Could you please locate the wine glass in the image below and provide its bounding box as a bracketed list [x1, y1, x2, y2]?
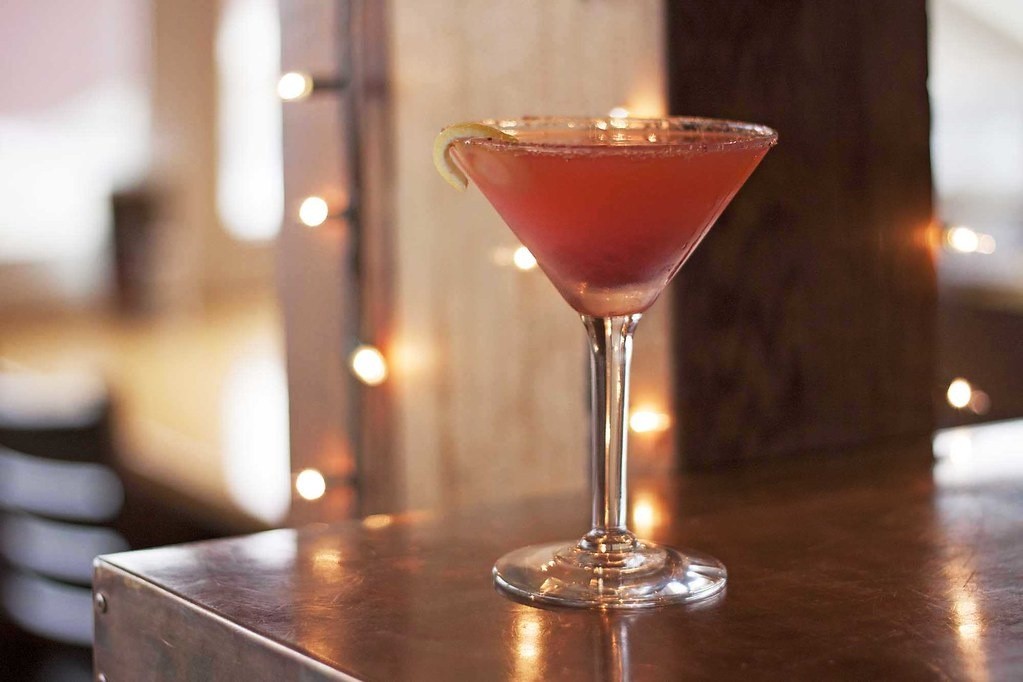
[442, 115, 781, 607]
